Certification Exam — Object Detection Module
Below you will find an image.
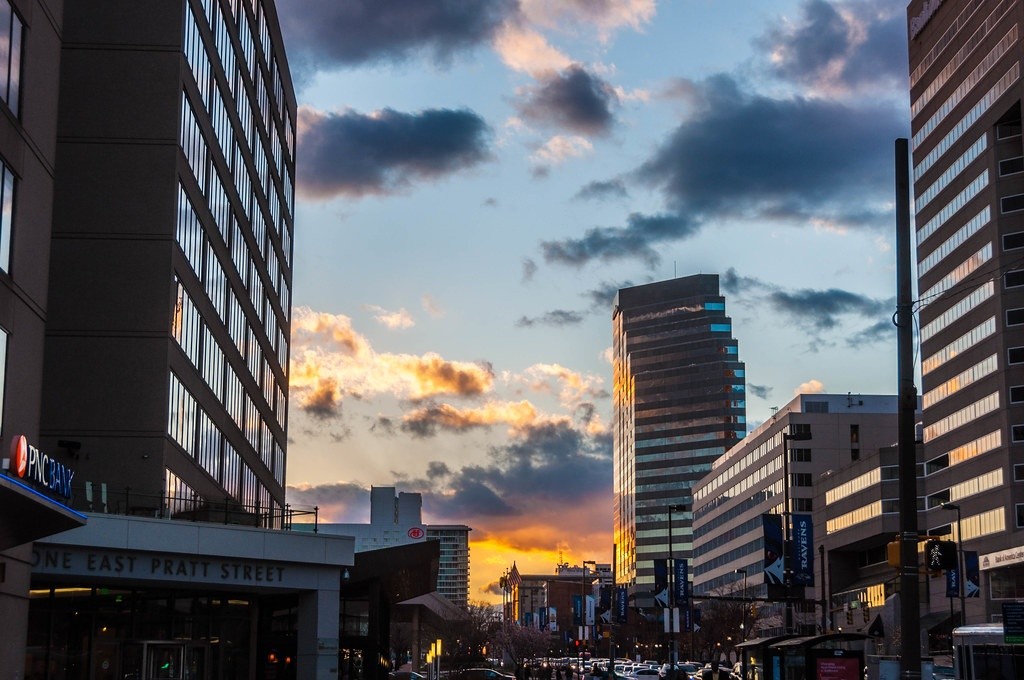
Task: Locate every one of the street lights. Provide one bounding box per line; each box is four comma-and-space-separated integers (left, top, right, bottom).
781, 430, 814, 632
431, 642, 436, 680
666, 505, 686, 680
436, 638, 442, 680
939, 503, 966, 626
735, 568, 748, 643
426, 653, 430, 680
581, 560, 596, 670
429, 649, 432, 680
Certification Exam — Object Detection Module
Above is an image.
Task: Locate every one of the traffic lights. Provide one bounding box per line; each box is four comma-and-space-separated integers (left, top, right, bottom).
925, 539, 951, 571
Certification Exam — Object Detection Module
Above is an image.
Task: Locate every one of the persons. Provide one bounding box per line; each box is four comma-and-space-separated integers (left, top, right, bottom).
514, 662, 601, 680
702, 661, 729, 680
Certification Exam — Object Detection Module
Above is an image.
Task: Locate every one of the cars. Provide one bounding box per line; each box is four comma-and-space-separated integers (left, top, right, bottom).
460, 667, 515, 680
488, 656, 764, 680
